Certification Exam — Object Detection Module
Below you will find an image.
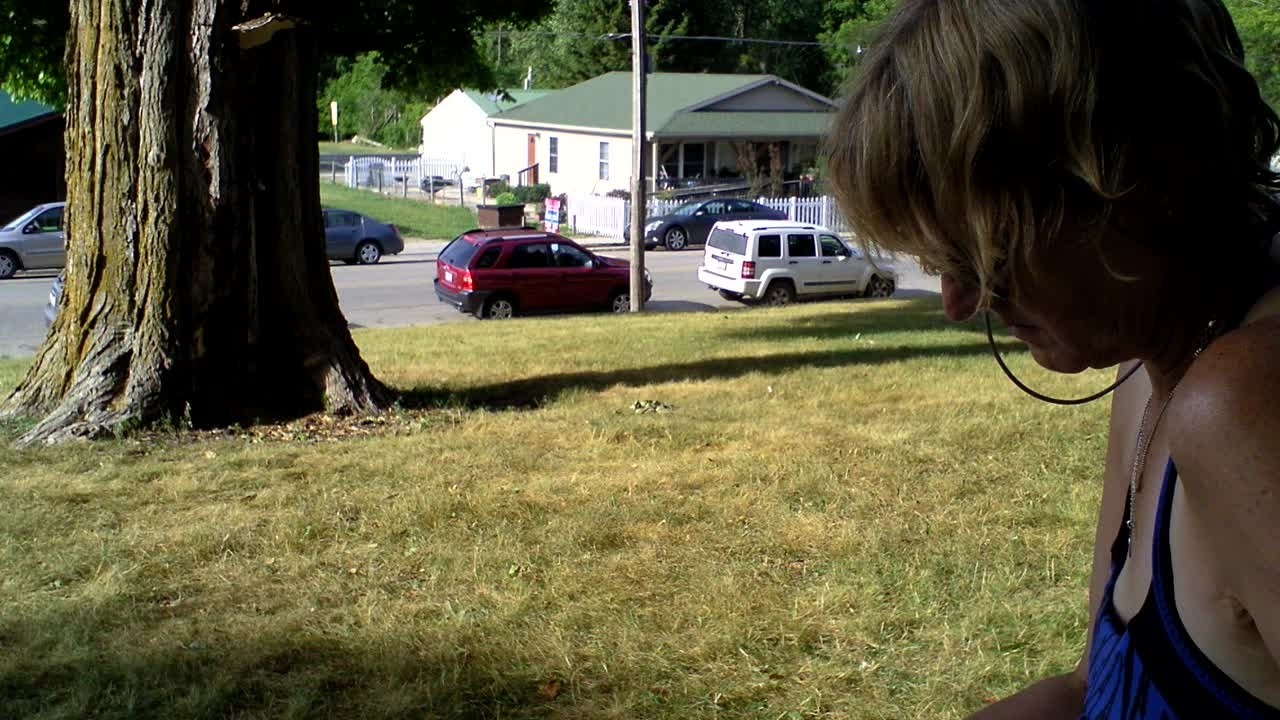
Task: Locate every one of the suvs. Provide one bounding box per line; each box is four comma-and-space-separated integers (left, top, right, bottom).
697, 219, 897, 306
0, 202, 67, 280
432, 225, 653, 320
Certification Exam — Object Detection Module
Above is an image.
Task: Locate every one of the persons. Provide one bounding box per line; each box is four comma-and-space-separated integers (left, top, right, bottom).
811, 0, 1280, 720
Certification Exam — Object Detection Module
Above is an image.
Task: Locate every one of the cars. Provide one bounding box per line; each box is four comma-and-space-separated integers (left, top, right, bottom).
322, 209, 403, 265
624, 199, 788, 251
